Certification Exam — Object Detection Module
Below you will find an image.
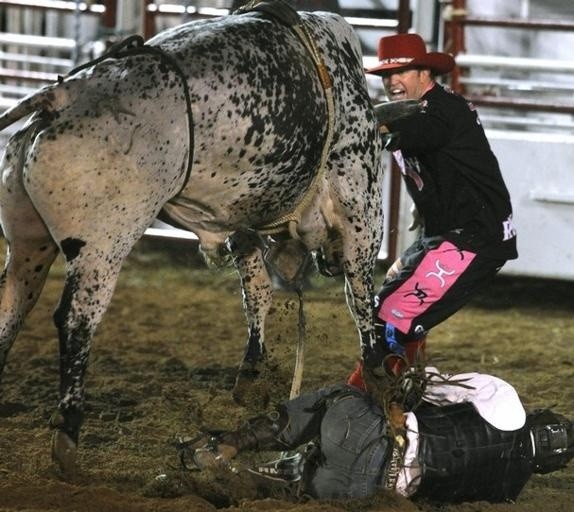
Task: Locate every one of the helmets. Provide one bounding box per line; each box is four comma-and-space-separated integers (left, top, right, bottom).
526, 409, 574, 475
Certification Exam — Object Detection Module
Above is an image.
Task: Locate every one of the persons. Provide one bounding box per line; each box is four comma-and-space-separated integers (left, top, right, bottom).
177, 358, 573, 508
343, 33, 518, 400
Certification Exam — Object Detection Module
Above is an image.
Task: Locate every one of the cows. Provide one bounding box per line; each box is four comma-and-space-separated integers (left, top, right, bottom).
0, 12, 385, 486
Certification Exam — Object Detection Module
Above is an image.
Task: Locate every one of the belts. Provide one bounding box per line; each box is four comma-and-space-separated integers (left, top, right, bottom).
376, 428, 407, 495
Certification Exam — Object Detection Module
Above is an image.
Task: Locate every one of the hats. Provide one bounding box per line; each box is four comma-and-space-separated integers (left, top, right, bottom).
366, 34, 456, 74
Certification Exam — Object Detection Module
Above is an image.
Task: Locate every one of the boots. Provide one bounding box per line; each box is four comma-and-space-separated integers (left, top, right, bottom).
148, 403, 294, 487
